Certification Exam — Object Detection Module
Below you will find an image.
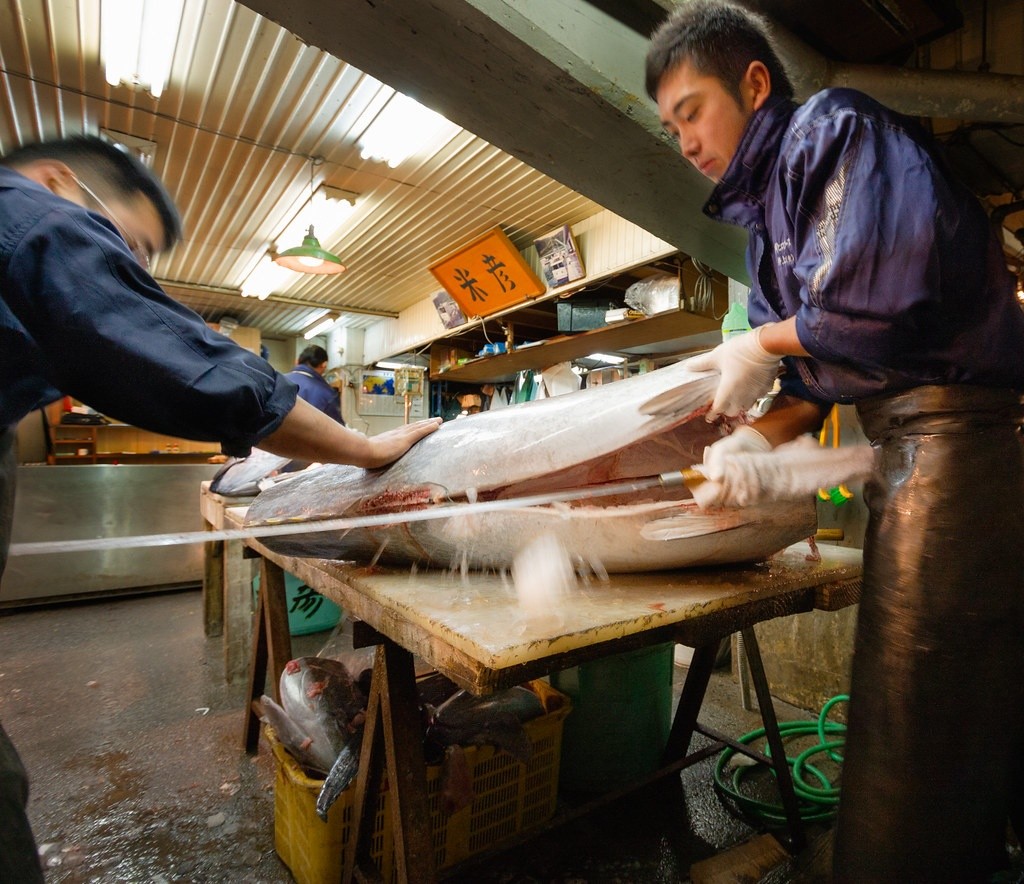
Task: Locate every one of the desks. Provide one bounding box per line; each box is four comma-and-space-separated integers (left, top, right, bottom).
222, 506, 866, 883
198, 478, 259, 687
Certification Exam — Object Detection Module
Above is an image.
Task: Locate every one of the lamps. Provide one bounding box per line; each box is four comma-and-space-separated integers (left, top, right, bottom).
217, 317, 240, 337
237, 184, 360, 301
300, 313, 341, 340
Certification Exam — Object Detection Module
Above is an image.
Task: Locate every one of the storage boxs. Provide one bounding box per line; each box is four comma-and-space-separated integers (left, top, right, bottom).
264, 666, 572, 884
253, 570, 344, 638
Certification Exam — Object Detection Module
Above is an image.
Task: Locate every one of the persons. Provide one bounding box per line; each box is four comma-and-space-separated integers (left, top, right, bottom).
642, 0, 1023, 882
0, 134, 443, 884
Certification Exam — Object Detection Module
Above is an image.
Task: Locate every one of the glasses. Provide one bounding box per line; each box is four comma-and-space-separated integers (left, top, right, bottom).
71, 175, 153, 275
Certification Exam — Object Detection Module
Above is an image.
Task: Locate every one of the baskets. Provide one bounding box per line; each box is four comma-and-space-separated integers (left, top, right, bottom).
265, 678, 574, 884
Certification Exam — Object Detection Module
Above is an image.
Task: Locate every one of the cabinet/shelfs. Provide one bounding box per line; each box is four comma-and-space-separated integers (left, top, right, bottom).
49, 396, 225, 467
428, 251, 734, 384
